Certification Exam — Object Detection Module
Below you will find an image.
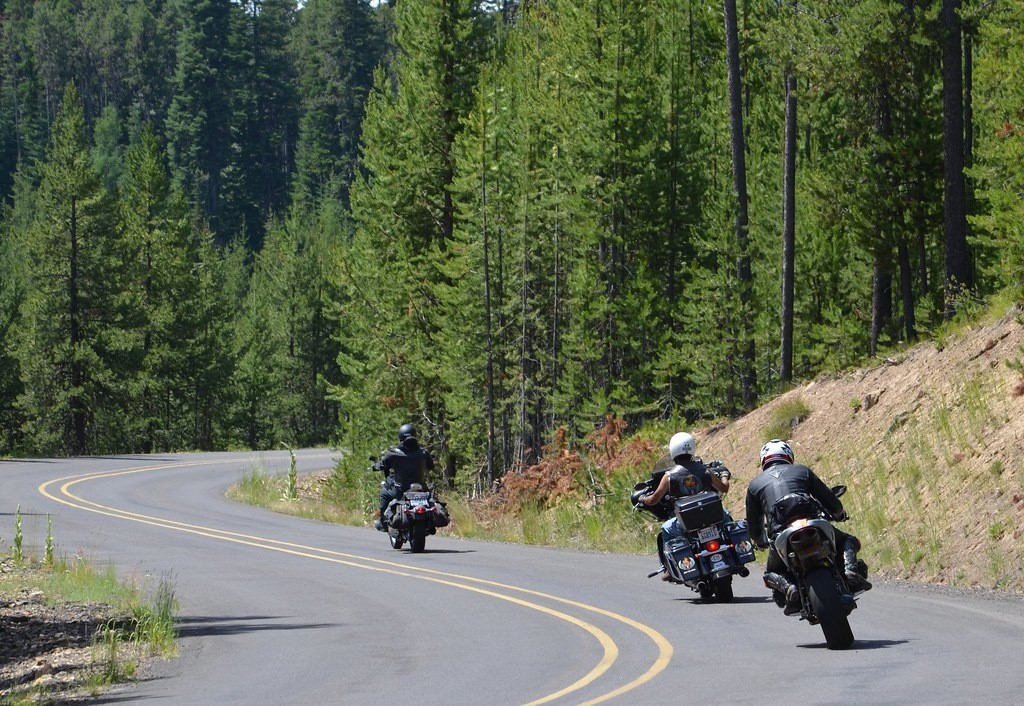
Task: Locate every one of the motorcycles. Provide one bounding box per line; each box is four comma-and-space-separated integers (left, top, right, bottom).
367, 454, 450, 553
752, 485, 873, 650
631, 454, 756, 601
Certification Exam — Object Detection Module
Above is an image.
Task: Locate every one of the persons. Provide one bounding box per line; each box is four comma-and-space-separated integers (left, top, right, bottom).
746, 439, 872, 616
637, 432, 732, 582
370, 424, 436, 535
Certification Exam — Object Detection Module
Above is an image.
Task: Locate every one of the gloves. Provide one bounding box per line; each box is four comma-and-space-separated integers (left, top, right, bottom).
717, 466, 730, 479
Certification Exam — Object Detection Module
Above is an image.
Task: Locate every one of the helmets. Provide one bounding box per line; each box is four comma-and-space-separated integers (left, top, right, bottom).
759, 439, 794, 471
397, 425, 417, 441
669, 432, 695, 460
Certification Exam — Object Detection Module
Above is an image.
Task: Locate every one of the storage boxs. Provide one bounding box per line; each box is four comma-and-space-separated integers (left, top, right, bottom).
664, 536, 700, 582
675, 490, 724, 530
725, 519, 756, 565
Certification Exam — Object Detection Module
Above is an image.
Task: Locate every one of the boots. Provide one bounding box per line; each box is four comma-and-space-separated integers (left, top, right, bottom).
766, 572, 802, 616
841, 550, 872, 593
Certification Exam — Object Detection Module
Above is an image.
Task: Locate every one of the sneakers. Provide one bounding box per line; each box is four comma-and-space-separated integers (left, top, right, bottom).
661, 572, 673, 581
375, 521, 388, 533
